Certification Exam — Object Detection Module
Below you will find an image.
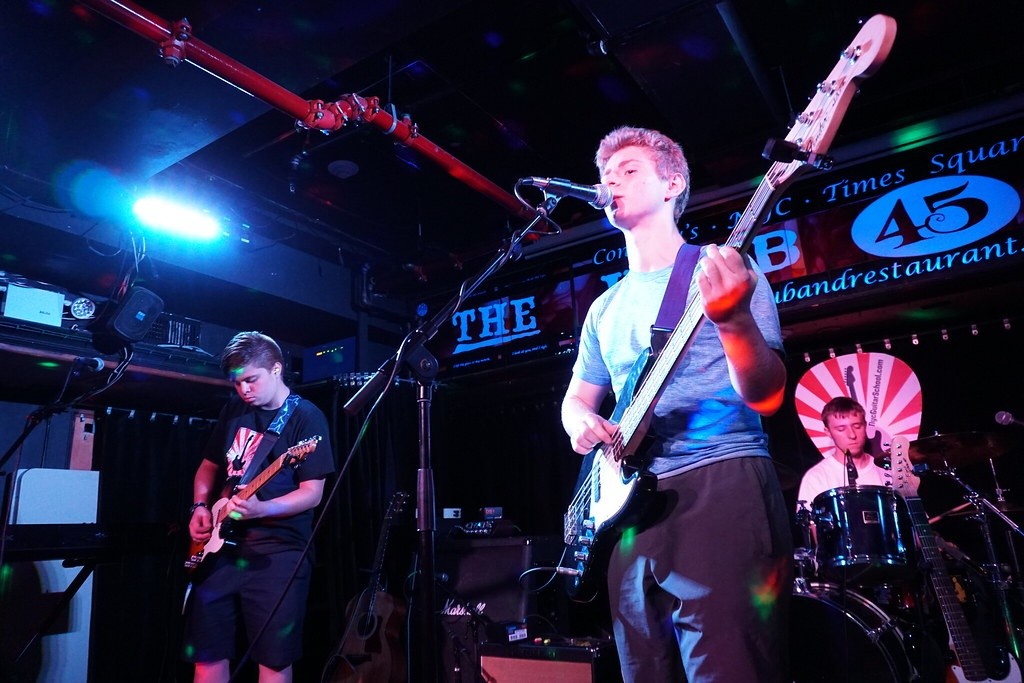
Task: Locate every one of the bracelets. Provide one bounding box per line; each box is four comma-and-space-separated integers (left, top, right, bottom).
189, 502, 209, 517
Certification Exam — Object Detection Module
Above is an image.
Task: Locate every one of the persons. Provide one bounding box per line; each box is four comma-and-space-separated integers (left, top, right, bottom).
188, 332, 334, 683
796, 396, 958, 560
561, 128, 787, 683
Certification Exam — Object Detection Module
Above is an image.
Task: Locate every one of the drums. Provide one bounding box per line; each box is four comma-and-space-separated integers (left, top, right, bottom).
812, 483, 912, 576
786, 582, 919, 683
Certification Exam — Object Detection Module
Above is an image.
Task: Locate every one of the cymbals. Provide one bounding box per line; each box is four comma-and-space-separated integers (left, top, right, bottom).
947, 499, 1023, 519
907, 428, 1023, 468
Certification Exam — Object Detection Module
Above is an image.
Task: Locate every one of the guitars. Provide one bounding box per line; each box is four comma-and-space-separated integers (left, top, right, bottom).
184, 435, 323, 575
321, 489, 411, 683
883, 441, 1023, 683
550, 13, 898, 605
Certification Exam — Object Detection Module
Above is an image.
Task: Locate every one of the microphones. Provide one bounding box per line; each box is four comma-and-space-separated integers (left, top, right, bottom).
845, 449, 858, 480
77, 356, 104, 372
995, 411, 1024, 427
519, 175, 613, 210
418, 569, 449, 583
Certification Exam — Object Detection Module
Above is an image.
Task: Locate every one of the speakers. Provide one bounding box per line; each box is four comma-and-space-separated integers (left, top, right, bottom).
433, 534, 565, 683
105, 286, 164, 344
478, 638, 624, 683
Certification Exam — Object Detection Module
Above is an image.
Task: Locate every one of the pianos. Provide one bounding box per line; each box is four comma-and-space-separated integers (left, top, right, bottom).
0, 523, 177, 564
